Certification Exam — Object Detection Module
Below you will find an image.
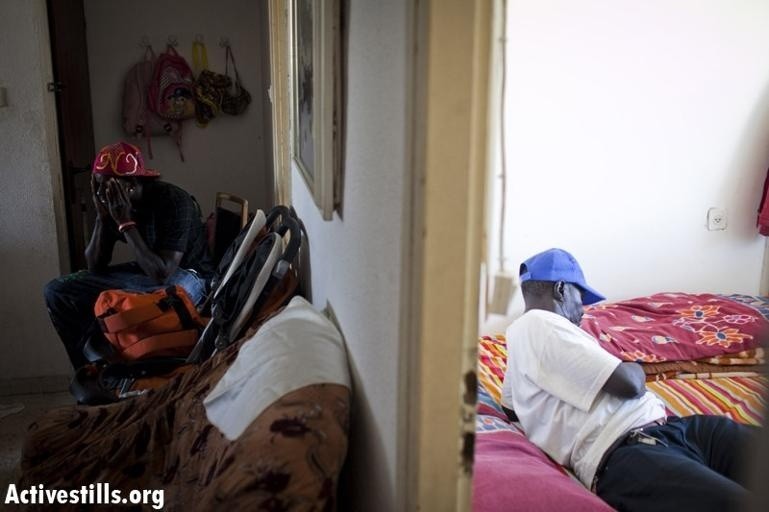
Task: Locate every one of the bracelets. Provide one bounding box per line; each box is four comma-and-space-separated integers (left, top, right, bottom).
118, 220, 138, 234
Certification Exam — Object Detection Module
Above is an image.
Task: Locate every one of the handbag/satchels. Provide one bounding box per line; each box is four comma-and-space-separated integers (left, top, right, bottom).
222, 87, 250, 114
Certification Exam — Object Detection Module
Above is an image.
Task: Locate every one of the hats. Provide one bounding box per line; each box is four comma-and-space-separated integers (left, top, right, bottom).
92, 142, 160, 176
519, 249, 606, 304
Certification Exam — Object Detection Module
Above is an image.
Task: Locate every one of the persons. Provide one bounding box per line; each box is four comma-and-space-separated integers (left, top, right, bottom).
499, 248, 769, 512
42, 140, 218, 377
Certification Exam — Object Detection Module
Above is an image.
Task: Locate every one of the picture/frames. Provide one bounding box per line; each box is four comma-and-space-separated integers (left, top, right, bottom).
292, 0, 341, 220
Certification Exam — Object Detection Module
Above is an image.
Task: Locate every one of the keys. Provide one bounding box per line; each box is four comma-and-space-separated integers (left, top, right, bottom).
626, 431, 657, 448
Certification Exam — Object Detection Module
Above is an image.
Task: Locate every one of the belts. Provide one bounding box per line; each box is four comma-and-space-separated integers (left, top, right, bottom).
592, 417, 666, 494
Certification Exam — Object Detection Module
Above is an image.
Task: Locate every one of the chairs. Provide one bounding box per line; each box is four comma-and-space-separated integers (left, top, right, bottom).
5, 304, 350, 511
214, 193, 249, 272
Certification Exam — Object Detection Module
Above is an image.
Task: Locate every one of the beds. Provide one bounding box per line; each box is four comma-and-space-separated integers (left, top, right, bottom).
473, 294, 769, 512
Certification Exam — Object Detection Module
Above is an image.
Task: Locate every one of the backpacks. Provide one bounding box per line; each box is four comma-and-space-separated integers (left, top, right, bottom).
149, 53, 196, 120
123, 44, 179, 137
95, 288, 205, 366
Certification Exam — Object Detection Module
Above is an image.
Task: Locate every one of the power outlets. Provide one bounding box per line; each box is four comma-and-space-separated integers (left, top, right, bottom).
708, 208, 727, 231
